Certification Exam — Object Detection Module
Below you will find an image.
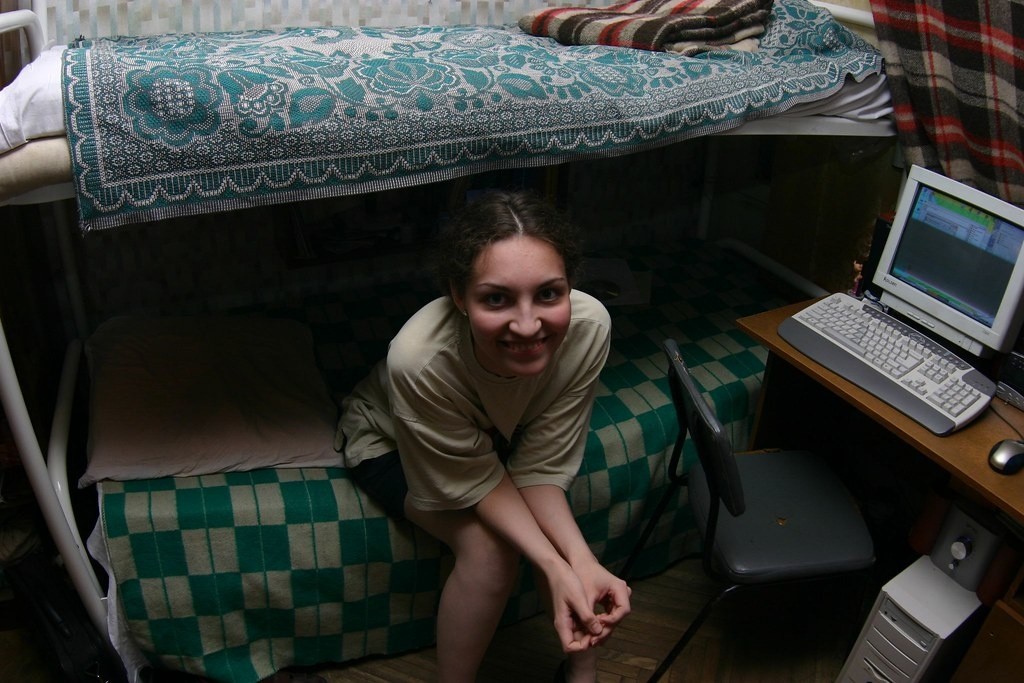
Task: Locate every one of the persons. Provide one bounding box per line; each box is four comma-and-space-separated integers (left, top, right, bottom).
330, 191, 632, 683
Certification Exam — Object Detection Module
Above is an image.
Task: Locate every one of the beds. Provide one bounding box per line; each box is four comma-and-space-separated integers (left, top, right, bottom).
0, 9, 894, 683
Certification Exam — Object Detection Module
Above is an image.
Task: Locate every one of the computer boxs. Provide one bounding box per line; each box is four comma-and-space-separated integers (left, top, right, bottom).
835, 553, 988, 683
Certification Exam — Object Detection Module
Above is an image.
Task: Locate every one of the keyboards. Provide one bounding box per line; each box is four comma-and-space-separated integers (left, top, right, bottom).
778, 293, 997, 437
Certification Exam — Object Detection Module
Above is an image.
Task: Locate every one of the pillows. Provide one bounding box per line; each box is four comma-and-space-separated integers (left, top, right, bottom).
78, 314, 346, 489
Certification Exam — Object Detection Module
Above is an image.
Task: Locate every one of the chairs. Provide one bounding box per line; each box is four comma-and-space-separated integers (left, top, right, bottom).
618, 341, 879, 683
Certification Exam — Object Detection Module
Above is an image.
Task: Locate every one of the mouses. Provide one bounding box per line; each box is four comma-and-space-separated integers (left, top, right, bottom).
987, 438, 1024, 475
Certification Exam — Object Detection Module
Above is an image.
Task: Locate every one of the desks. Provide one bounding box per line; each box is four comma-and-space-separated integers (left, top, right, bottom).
733, 289, 1024, 683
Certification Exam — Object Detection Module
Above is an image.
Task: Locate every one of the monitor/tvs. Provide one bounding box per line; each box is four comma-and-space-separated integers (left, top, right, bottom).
871, 164, 1024, 357
